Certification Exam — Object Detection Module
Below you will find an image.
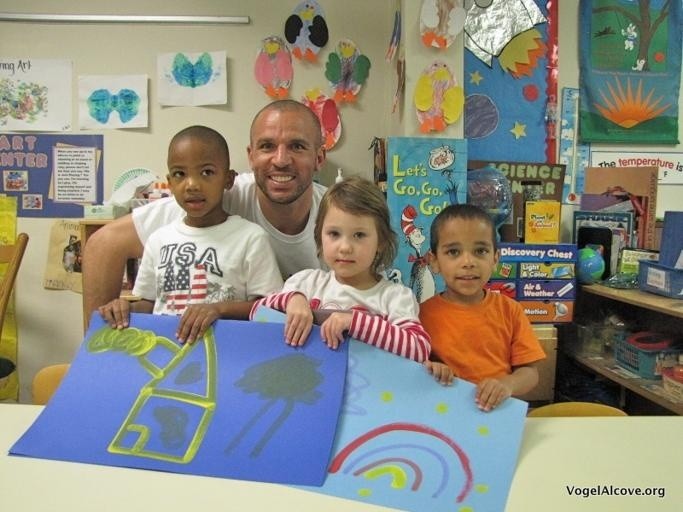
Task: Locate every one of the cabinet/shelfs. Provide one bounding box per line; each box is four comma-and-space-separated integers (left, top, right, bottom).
552, 272, 682, 415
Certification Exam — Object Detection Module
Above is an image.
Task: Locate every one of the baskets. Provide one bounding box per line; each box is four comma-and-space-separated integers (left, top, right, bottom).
611, 329, 683, 404
636, 259, 683, 299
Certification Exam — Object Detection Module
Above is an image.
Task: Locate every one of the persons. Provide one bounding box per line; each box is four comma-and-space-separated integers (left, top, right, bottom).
416, 204, 549, 414
246, 173, 432, 365
77, 100, 390, 342
97, 123, 285, 345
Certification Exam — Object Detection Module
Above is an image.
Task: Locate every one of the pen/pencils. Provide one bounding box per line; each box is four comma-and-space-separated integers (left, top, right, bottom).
130, 174, 159, 200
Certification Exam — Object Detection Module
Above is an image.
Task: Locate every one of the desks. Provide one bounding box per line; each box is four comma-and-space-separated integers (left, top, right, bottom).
1, 400, 683, 511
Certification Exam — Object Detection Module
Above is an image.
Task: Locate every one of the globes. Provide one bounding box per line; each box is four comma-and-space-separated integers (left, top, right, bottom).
574, 244, 605, 285
